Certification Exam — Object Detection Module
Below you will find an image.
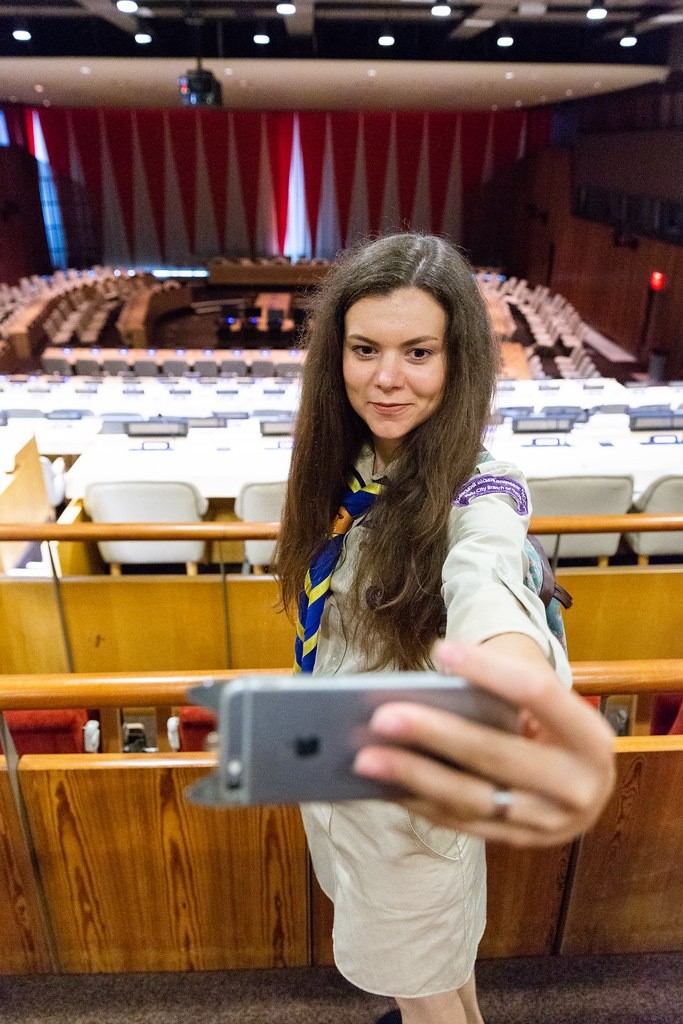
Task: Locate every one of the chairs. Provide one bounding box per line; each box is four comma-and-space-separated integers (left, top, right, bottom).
0, 256, 683, 575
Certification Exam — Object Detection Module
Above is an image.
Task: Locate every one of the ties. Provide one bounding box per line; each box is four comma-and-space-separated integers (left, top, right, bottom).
294, 468, 395, 674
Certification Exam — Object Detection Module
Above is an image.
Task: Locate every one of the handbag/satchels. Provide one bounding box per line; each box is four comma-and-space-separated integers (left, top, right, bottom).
525, 530, 571, 649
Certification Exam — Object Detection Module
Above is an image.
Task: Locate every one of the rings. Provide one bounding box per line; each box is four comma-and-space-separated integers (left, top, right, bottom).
489, 785, 509, 824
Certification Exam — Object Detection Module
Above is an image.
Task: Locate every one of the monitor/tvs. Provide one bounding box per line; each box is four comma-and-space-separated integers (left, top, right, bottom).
220, 303, 306, 325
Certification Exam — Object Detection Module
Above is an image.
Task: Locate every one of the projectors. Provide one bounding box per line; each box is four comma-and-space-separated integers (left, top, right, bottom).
178, 70, 222, 108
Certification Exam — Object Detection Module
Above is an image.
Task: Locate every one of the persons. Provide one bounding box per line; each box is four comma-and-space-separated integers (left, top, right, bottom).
273, 235, 618, 1024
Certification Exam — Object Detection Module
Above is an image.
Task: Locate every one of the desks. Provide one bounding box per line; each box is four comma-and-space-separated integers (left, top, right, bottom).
500, 342, 532, 380
115, 283, 192, 349
481, 293, 509, 334
8, 297, 56, 359
208, 264, 334, 284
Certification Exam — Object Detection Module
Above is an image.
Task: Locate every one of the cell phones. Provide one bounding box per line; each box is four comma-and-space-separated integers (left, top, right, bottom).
186, 673, 520, 810
